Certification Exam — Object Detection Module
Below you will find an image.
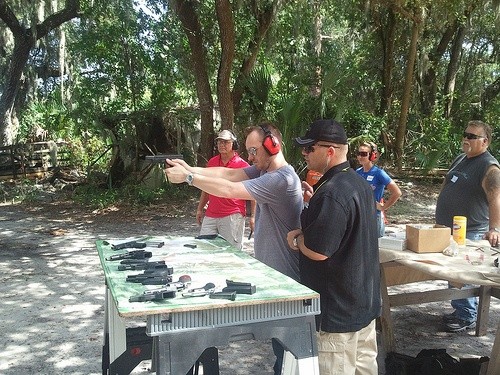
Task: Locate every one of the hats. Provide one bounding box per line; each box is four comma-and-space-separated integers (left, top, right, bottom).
295, 120, 348, 144
215, 130, 236, 142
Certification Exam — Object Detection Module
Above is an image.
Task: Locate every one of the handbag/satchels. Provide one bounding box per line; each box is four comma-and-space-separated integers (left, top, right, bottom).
386, 348, 463, 375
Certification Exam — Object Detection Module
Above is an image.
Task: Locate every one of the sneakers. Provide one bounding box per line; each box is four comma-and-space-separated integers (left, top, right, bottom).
443, 312, 457, 321
448, 319, 476, 333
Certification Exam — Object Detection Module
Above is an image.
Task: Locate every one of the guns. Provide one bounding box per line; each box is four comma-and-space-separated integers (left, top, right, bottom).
146, 153, 184, 166
106, 240, 258, 303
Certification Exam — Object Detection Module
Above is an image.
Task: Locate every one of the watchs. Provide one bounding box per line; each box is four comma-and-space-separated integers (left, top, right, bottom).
186, 173, 193, 186
293, 234, 301, 249
489, 228, 500, 232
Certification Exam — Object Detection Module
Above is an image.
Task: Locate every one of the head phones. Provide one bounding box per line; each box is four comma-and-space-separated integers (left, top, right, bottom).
214, 129, 240, 151
258, 125, 281, 156
367, 143, 380, 162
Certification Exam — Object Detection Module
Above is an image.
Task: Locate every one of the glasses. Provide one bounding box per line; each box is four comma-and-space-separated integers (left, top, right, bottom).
247, 144, 263, 156
217, 141, 232, 145
305, 144, 340, 153
463, 132, 487, 139
357, 152, 371, 157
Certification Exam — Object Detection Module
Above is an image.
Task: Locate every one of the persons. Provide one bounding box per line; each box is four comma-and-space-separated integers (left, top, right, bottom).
304, 170, 323, 207
356, 141, 403, 239
196, 130, 256, 251
436, 120, 500, 333
163, 120, 304, 375
286, 120, 383, 375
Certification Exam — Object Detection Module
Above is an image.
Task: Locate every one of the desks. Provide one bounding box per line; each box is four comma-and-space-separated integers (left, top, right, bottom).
95, 233, 322, 375
379, 222, 500, 375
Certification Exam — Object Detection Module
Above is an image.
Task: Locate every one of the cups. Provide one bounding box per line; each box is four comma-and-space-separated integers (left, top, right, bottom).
453, 216, 467, 248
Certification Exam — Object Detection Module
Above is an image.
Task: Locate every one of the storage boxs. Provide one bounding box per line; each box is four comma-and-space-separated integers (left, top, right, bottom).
378, 223, 451, 253
